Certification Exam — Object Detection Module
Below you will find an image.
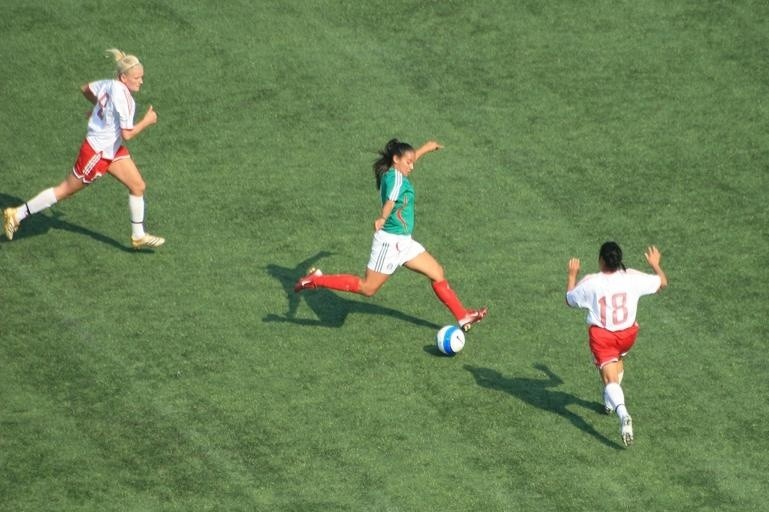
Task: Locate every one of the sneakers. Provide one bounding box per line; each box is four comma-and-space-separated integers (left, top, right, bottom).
603, 402, 615, 415
293, 267, 322, 292
4, 207, 20, 240
130, 233, 165, 251
457, 307, 488, 332
620, 415, 634, 446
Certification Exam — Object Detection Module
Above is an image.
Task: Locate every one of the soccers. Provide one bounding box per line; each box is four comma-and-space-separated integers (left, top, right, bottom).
436, 325, 465, 357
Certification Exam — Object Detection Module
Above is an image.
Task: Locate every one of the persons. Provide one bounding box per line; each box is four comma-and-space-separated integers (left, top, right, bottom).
564, 240, 669, 447
294, 139, 488, 333
3, 47, 167, 249
